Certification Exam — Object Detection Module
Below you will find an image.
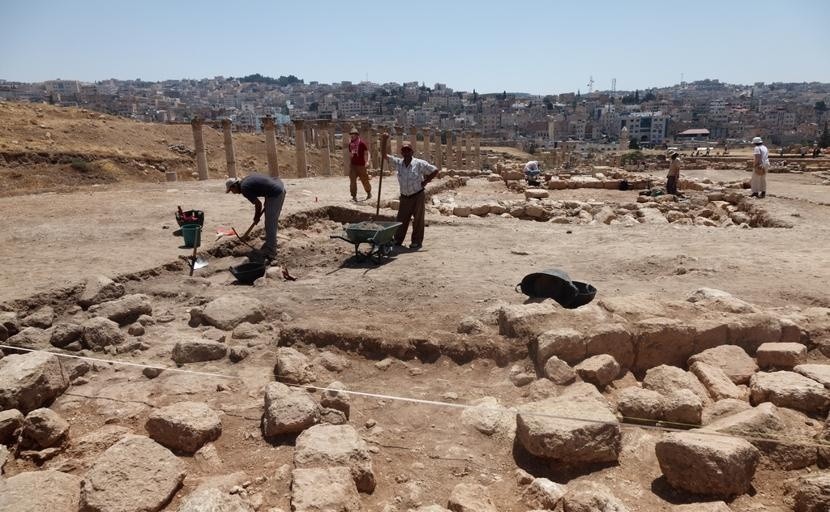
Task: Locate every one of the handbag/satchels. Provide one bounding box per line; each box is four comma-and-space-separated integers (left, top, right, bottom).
755, 162, 765, 175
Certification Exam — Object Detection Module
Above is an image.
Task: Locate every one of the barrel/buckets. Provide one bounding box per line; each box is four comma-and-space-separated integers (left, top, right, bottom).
182, 224, 201, 248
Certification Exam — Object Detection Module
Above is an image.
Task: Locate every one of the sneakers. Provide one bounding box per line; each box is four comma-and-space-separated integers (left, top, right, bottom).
349, 193, 372, 202
389, 239, 400, 246
410, 242, 422, 250
749, 192, 765, 198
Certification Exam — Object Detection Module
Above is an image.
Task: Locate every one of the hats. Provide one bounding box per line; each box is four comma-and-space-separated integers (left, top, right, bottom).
668, 149, 676, 156
750, 137, 763, 145
226, 178, 239, 194
349, 128, 360, 136
401, 144, 414, 151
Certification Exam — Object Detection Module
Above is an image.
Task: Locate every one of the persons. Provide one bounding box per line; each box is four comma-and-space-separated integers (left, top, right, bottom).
748, 137, 770, 198
690, 143, 822, 160
347, 127, 372, 202
226, 174, 286, 260
381, 129, 439, 250
665, 149, 682, 195
523, 160, 541, 181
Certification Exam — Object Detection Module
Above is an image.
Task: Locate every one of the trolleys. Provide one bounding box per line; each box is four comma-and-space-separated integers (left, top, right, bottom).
329, 220, 404, 265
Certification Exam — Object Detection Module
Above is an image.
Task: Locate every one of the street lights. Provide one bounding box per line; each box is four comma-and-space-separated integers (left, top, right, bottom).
500, 110, 505, 127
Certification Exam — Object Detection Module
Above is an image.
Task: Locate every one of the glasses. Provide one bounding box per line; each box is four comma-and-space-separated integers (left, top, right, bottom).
402, 149, 411, 152
